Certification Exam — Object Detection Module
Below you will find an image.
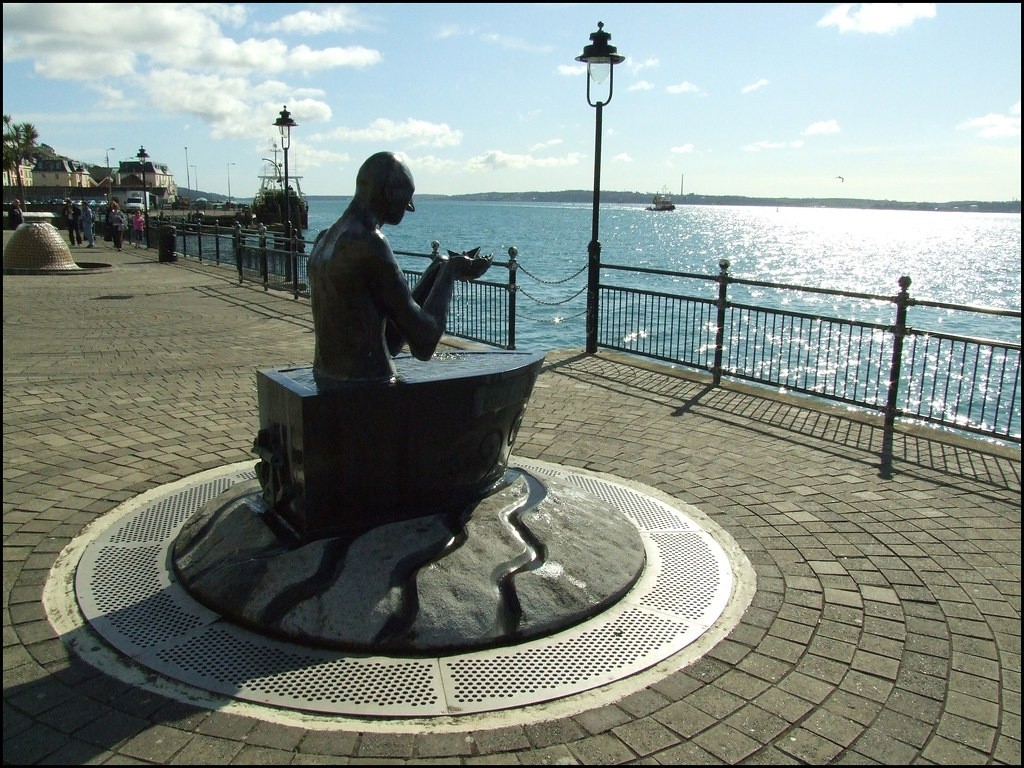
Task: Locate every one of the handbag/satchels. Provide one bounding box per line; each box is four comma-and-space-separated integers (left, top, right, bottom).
118, 224, 128, 231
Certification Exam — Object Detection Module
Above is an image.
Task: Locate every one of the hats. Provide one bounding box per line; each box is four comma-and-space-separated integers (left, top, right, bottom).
64, 199, 71, 204
82, 201, 89, 206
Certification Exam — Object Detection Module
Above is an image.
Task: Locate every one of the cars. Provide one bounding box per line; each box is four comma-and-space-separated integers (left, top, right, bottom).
95, 200, 107, 206
87, 199, 97, 206
71, 199, 82, 205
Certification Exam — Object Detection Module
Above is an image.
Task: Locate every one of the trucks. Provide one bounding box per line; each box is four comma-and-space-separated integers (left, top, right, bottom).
123, 191, 150, 214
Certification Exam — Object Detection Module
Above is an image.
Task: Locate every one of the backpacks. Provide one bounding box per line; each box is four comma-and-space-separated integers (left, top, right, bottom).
88, 207, 96, 223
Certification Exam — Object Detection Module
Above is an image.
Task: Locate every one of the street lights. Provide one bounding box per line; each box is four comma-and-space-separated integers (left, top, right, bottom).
227, 162, 236, 205
273, 103, 302, 282
575, 19, 625, 355
106, 147, 115, 199
135, 145, 150, 248
189, 164, 198, 196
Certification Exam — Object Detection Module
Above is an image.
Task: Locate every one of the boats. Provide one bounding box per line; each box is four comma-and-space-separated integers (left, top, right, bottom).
644, 192, 677, 211
248, 141, 310, 230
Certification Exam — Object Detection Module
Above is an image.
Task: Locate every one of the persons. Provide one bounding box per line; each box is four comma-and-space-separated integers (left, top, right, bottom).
62, 197, 143, 253
9, 199, 23, 230
306, 151, 493, 383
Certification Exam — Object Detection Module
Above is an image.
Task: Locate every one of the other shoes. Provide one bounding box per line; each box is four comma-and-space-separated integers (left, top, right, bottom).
118, 248, 121, 252
69, 244, 76, 247
78, 244, 82, 248
86, 245, 95, 248
